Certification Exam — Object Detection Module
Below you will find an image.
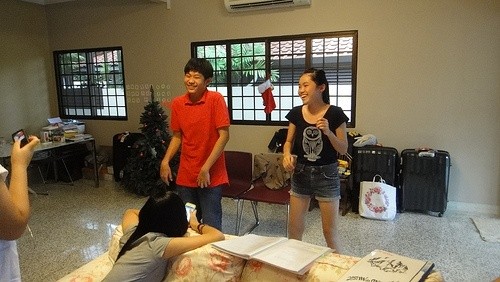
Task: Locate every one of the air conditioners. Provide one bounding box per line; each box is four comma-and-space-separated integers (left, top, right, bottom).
224, 0, 311, 13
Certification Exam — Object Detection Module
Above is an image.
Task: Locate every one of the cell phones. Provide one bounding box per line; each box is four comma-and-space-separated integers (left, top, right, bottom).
185, 202, 197, 223
11, 129, 29, 148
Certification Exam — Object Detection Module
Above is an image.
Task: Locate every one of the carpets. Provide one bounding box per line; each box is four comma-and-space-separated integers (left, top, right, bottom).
470, 216, 500, 243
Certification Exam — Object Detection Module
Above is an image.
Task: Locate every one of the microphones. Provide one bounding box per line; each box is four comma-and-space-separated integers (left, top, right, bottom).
250, 172, 268, 186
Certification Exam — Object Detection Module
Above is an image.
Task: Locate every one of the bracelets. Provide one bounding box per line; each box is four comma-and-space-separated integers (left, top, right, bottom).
197, 224, 205, 234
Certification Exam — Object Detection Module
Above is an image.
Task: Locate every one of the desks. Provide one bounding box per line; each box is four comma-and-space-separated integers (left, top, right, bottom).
0, 134, 100, 188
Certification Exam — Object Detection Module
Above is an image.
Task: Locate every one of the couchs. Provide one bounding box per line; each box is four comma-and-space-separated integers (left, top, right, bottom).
59, 226, 446, 282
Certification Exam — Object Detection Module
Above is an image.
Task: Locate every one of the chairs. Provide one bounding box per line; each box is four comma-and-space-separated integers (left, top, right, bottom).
221, 129, 362, 239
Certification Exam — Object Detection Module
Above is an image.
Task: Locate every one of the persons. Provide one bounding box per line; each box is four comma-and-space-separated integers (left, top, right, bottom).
282, 68, 349, 253
102, 190, 225, 282
160, 58, 231, 233
0, 135, 41, 282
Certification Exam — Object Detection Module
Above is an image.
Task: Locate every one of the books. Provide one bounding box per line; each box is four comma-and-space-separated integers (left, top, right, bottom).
339, 249, 436, 282
212, 233, 335, 276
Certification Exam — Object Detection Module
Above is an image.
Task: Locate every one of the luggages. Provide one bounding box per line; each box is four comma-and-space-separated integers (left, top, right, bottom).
112, 133, 146, 180
400, 148, 451, 216
350, 146, 402, 213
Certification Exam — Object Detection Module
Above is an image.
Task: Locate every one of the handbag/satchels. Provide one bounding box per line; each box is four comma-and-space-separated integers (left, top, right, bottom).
359, 174, 398, 221
